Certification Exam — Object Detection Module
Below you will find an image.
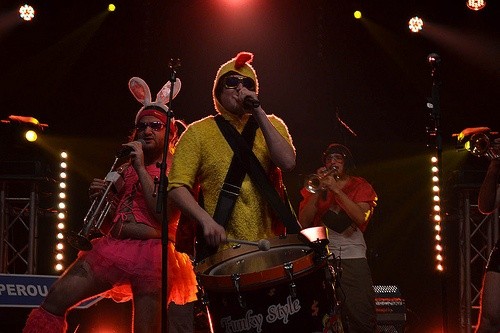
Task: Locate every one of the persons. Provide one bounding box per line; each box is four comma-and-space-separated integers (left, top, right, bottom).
22, 102, 198, 333
478, 138, 500, 333
167, 52, 295, 267
299, 144, 378, 333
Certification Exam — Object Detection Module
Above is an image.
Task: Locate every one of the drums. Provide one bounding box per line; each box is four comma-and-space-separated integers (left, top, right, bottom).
192, 234, 346, 333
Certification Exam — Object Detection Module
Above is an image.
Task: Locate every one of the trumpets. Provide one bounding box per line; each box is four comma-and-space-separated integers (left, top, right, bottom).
65, 127, 140, 252
303, 166, 341, 193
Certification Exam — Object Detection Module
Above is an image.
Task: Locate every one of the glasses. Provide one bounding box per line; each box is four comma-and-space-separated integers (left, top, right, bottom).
135, 122, 165, 131
325, 156, 345, 164
222, 76, 255, 90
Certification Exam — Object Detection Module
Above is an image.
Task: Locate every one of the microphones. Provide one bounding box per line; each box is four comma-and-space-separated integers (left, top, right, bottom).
243, 95, 260, 108
427, 53, 440, 65
